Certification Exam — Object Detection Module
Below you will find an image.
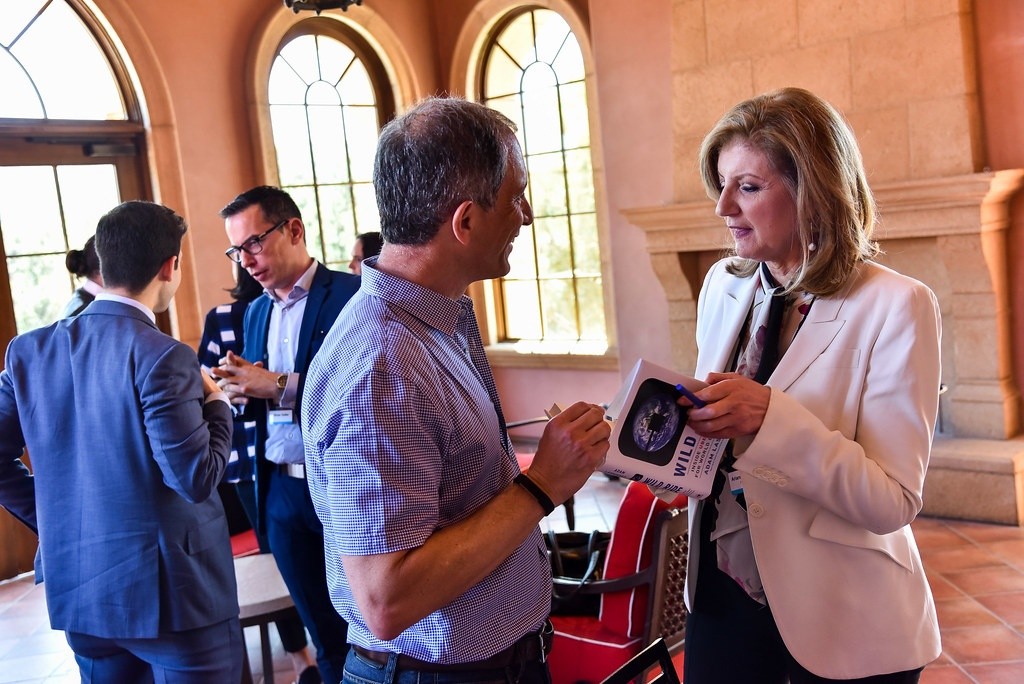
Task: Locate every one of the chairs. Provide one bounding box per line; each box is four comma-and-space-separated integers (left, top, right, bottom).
546, 476, 691, 684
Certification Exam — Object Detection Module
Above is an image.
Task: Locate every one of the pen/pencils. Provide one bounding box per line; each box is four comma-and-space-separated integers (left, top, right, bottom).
676, 382, 706, 409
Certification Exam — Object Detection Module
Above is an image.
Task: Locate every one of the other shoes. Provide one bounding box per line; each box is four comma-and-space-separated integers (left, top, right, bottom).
299, 666, 322, 684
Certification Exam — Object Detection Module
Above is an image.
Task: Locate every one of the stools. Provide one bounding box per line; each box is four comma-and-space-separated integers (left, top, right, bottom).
224, 554, 298, 684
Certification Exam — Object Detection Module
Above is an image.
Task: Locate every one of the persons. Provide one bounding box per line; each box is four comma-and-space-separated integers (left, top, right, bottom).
65, 185, 389, 684
0, 201, 244, 684
301, 95, 611, 684
647, 87, 943, 684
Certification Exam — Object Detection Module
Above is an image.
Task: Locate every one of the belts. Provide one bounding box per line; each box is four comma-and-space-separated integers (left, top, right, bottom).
282, 463, 307, 479
354, 618, 554, 672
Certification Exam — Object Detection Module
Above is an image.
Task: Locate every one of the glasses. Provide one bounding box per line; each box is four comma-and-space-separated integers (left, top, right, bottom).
226, 220, 287, 263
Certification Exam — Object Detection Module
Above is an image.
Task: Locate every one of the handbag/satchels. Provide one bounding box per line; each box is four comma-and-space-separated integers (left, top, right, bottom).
541, 531, 611, 617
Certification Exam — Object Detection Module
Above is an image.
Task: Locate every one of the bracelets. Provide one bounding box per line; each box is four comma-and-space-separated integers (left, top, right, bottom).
513, 474, 555, 516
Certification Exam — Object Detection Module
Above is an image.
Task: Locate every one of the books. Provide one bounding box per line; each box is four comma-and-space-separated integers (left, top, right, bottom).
544, 357, 730, 500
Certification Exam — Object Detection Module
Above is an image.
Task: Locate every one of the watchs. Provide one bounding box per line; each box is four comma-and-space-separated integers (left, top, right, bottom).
277, 373, 288, 398
